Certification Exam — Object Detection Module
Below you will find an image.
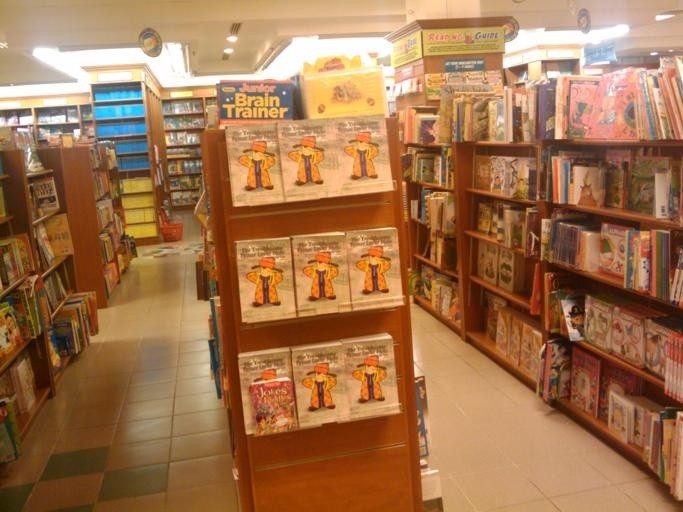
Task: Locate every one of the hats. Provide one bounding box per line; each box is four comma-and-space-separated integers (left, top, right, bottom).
307, 363, 336, 379
568, 305, 585, 317
348, 131, 379, 148
356, 355, 387, 372
244, 141, 275, 156
306, 252, 338, 267
252, 257, 284, 272
360, 246, 391, 262
292, 136, 324, 151
253, 369, 279, 382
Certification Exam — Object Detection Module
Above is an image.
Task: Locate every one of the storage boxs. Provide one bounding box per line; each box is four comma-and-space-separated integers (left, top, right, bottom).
296, 64, 389, 120
216, 79, 295, 130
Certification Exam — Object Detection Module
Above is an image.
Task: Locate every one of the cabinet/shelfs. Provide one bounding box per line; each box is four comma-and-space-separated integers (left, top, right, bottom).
91, 79, 170, 245
550, 84, 683, 501
0, 91, 94, 142
134, 82, 203, 246
26, 139, 131, 309
465, 99, 548, 390
396, 112, 465, 328
0, 147, 91, 443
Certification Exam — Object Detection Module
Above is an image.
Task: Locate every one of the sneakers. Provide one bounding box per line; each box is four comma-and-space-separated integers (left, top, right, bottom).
308, 296, 320, 303
327, 405, 336, 409
308, 406, 318, 412
351, 174, 361, 180
313, 179, 324, 185
369, 174, 378, 179
245, 184, 254, 192
358, 397, 367, 404
362, 289, 371, 295
376, 397, 386, 403
252, 302, 262, 306
271, 301, 282, 307
327, 294, 336, 300
263, 184, 275, 191
380, 288, 389, 293
295, 179, 305, 186
